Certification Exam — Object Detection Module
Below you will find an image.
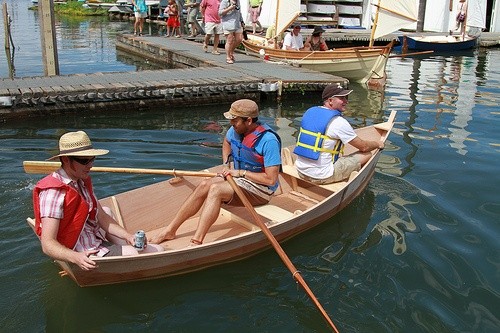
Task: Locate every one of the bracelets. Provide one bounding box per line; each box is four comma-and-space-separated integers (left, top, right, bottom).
243, 168, 248, 177
238, 169, 241, 177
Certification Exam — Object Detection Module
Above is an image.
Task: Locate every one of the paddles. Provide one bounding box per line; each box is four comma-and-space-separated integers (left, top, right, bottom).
333, 45, 390, 51
388, 50, 434, 59
21, 160, 226, 178
223, 167, 340, 333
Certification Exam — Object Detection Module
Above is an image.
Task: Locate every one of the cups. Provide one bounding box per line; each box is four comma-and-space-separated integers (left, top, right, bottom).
265, 54, 269, 60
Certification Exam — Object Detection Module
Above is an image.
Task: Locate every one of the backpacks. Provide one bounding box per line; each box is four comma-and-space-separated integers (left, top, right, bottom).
168, 5, 178, 16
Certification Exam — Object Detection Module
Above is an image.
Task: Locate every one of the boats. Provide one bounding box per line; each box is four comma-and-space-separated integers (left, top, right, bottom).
242, 12, 396, 84
398, 25, 481, 52
26, 108, 398, 287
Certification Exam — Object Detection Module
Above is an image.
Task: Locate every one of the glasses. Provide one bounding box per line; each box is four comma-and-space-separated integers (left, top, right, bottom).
69, 156, 96, 165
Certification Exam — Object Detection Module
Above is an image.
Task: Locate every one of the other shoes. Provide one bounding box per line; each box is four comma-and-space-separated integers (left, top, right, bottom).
251, 32, 256, 35
196, 33, 204, 37
139, 33, 143, 37
188, 34, 194, 38
203, 43, 208, 52
212, 50, 220, 55
133, 31, 137, 36
229, 54, 235, 62
180, 34, 185, 36
170, 34, 174, 37
259, 28, 264, 35
164, 34, 169, 38
226, 57, 233, 64
175, 34, 181, 38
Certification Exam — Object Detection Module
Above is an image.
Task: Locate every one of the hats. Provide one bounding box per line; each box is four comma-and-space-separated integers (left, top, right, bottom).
312, 26, 326, 34
321, 83, 353, 101
223, 99, 258, 119
45, 130, 109, 160
293, 23, 302, 30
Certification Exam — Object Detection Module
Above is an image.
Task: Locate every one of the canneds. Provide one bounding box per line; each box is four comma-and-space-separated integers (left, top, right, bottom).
135, 230, 145, 251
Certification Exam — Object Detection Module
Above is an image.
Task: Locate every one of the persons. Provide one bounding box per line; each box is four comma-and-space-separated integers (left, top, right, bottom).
218, 0, 246, 64
281, 23, 330, 52
248, 0, 265, 35
292, 83, 385, 185
148, 99, 282, 244
33, 130, 147, 273
131, 0, 222, 55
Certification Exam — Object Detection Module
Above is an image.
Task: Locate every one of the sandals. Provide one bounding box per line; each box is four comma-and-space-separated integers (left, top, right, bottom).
186, 238, 202, 247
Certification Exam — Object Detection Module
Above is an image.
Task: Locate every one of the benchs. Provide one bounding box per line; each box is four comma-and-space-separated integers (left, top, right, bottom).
183, 176, 294, 223
283, 165, 347, 192
